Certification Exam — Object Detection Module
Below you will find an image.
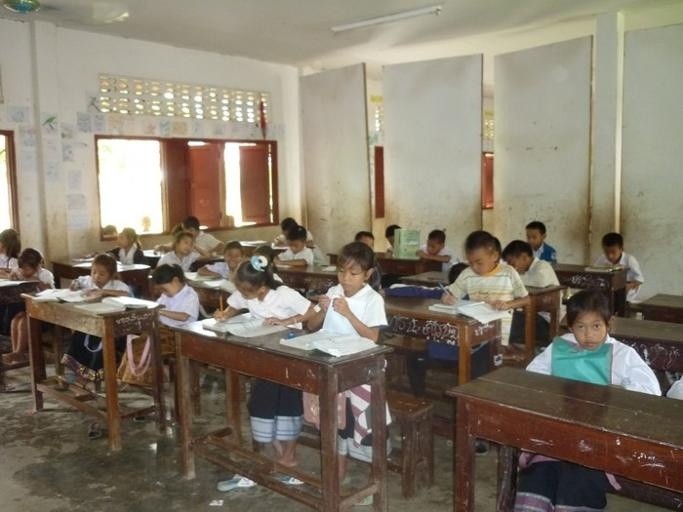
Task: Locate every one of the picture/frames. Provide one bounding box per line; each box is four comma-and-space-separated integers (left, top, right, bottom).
20, 291, 170, 453
0, 281, 39, 391
53, 253, 151, 299
444, 366, 682, 512
609, 315, 683, 373
238, 240, 263, 255
554, 262, 630, 315
400, 270, 451, 289
325, 250, 385, 268
143, 249, 161, 264
172, 317, 395, 511
636, 294, 682, 322
276, 266, 338, 294
377, 296, 501, 386
378, 257, 442, 276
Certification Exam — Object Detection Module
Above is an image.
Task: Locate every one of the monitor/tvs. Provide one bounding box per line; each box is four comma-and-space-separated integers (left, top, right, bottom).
584, 263, 623, 273
74, 296, 147, 315
427, 299, 510, 325
279, 328, 378, 358
202, 312, 290, 338
34, 289, 97, 302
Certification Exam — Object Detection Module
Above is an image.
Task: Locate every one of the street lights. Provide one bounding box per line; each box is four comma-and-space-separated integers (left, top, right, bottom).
551, 335, 613, 386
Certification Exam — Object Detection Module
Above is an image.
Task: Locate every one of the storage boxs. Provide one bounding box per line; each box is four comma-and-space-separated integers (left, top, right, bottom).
503, 341, 534, 370
387, 388, 435, 499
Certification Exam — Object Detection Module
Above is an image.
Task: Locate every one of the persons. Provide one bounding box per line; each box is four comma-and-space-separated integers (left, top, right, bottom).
392, 228, 421, 259
385, 262, 470, 398
106, 228, 146, 265
354, 230, 375, 252
196, 241, 243, 278
501, 239, 562, 342
213, 257, 316, 468
513, 290, 662, 512
273, 225, 314, 268
61, 254, 134, 383
2, 248, 56, 362
595, 232, 644, 304
525, 221, 558, 264
153, 225, 183, 254
439, 230, 530, 457
181, 216, 225, 256
306, 243, 393, 507
415, 229, 453, 273
385, 224, 402, 251
273, 216, 325, 264
116, 262, 200, 387
0, 229, 19, 280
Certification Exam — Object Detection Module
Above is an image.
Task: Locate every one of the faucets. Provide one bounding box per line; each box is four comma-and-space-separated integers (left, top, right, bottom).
87, 422, 102, 439
135, 413, 146, 422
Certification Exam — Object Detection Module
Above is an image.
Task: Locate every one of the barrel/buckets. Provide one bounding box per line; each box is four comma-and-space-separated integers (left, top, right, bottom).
358, 498, 373, 506
270, 471, 302, 485
217, 476, 256, 492
336, 474, 350, 495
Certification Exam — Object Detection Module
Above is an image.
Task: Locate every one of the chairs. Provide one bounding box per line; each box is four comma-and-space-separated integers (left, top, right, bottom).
476, 440, 489, 455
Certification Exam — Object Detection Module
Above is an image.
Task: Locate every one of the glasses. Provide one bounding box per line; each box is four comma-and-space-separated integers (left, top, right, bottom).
330, 4, 444, 35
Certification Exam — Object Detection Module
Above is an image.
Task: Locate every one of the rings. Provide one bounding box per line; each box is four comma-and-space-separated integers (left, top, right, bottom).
220, 295, 222, 312
438, 282, 458, 303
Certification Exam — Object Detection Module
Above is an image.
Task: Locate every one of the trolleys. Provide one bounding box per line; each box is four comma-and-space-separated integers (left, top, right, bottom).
0, 0, 61, 21
